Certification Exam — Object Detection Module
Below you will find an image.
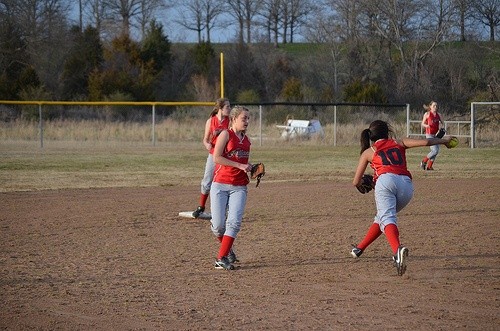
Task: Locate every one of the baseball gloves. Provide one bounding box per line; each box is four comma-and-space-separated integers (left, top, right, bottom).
247, 162, 266, 180
356, 175, 378, 194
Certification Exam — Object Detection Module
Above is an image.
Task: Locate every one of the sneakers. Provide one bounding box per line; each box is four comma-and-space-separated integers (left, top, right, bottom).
192, 207, 204, 218
228, 251, 235, 263
215, 257, 234, 270
350, 247, 363, 258
392, 246, 409, 275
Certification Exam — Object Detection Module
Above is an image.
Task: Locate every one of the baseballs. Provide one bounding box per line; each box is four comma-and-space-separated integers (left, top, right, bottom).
435, 128, 446, 138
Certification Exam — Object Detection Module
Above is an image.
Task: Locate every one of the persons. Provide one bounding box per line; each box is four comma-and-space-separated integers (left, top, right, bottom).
348, 119, 458, 275
192, 99, 232, 218
210, 105, 265, 270
421, 100, 445, 170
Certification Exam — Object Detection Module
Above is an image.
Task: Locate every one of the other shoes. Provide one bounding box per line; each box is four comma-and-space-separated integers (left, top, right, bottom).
421, 161, 427, 170
427, 167, 433, 170
